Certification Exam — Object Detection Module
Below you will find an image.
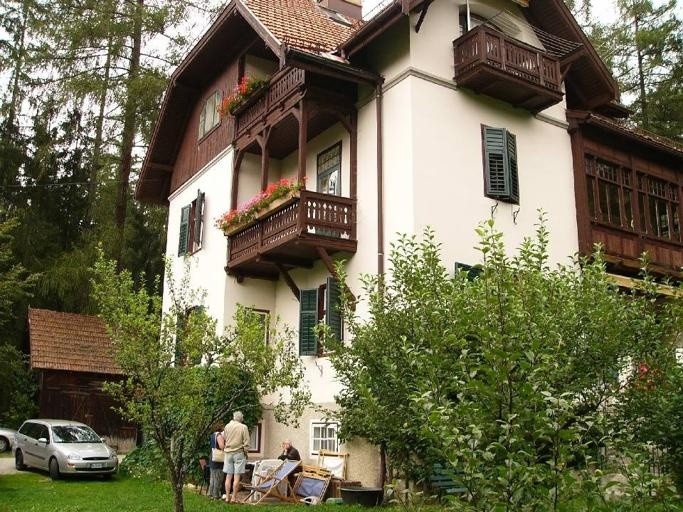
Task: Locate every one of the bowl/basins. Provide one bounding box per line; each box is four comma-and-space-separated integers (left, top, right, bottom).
339, 486, 384, 508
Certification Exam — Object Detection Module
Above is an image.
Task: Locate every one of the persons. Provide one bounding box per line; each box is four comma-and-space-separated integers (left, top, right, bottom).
276, 439, 302, 496
220, 410, 251, 504
206, 419, 226, 498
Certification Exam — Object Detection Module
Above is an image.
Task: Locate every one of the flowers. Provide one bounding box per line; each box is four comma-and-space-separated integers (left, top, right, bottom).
214, 71, 255, 118
214, 176, 299, 231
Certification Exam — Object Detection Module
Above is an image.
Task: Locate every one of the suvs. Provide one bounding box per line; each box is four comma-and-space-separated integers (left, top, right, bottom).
12, 417, 121, 484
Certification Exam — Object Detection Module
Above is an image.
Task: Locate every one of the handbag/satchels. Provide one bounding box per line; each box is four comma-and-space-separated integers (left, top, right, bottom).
210, 432, 225, 463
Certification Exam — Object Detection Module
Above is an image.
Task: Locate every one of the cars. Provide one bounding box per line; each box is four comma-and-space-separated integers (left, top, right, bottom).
0, 426, 19, 454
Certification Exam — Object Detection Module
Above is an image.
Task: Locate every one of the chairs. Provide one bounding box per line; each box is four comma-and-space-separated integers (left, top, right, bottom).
241, 458, 303, 505
